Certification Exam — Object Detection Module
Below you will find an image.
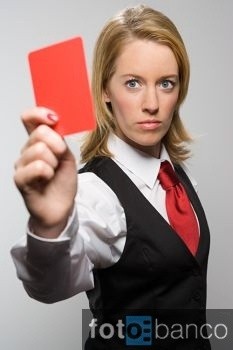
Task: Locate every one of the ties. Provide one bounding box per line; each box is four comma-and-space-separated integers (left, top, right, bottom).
157, 161, 199, 259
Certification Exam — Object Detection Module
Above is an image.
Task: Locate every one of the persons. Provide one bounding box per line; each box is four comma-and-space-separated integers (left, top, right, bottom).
11, 5, 212, 345
